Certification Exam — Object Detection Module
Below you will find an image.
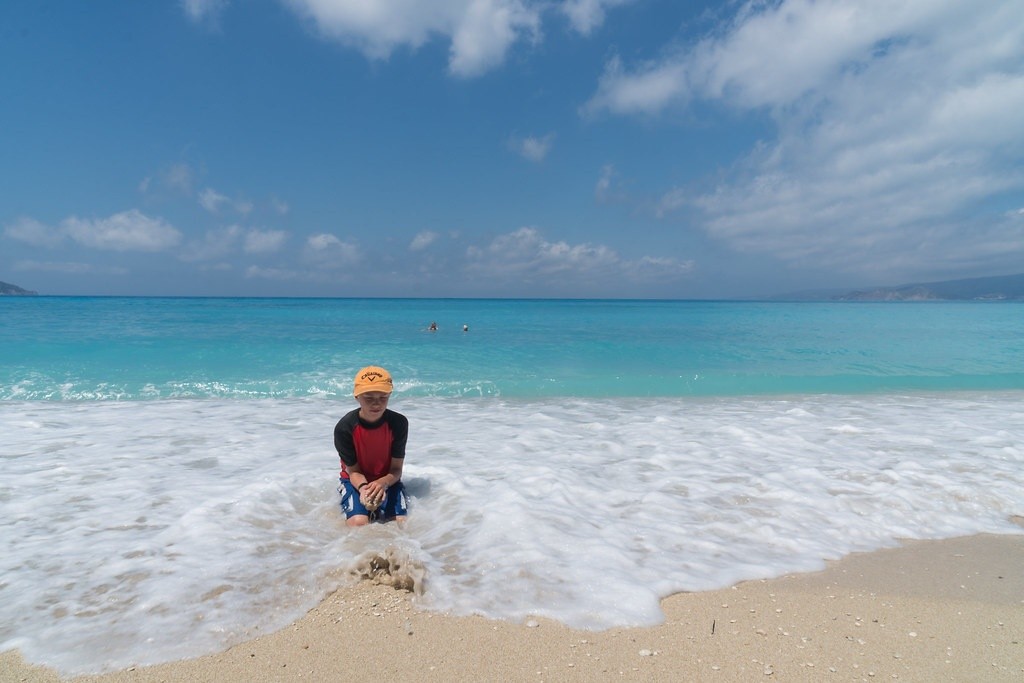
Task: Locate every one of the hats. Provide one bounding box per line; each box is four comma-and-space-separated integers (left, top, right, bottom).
354, 365, 393, 396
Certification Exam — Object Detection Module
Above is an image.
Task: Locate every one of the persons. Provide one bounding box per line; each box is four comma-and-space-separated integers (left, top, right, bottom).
334, 365, 409, 527
429, 321, 468, 331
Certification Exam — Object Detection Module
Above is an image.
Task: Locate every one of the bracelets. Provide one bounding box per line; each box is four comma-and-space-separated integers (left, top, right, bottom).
357, 483, 368, 491
